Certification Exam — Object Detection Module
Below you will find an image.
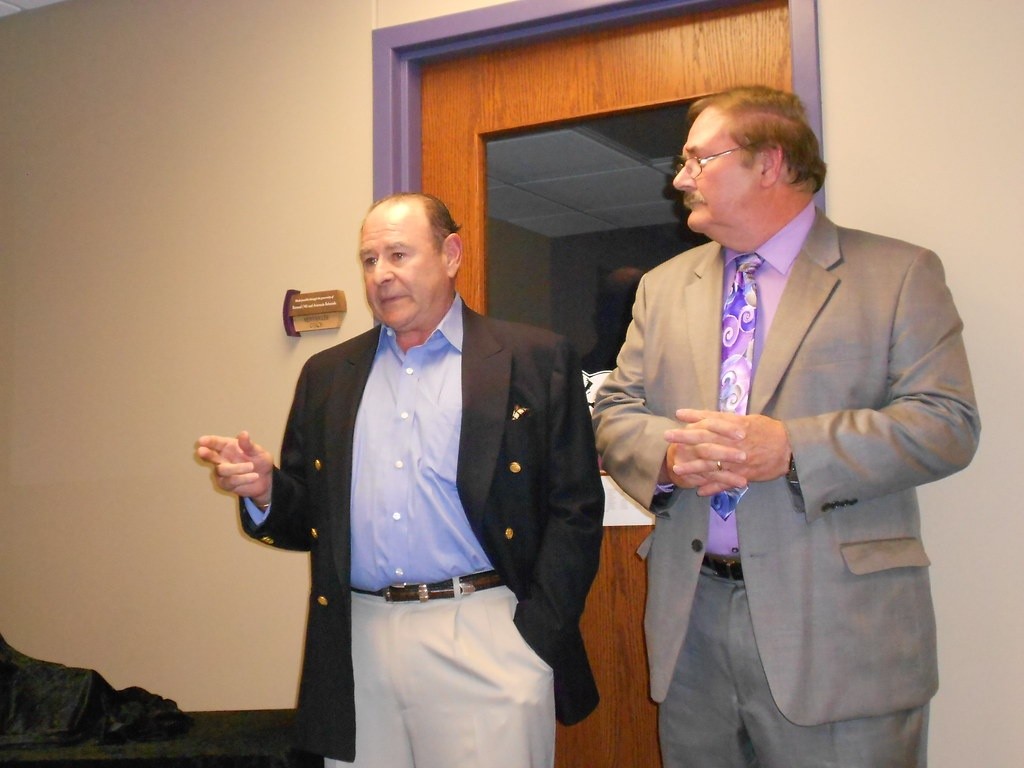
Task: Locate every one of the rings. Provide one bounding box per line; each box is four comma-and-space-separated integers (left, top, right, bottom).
717, 461, 722, 471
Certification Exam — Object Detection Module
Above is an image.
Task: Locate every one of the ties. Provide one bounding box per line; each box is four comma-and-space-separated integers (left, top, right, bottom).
708, 253, 765, 522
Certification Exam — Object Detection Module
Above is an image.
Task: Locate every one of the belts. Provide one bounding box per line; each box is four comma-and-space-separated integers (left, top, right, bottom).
349, 569, 505, 603
702, 555, 742, 582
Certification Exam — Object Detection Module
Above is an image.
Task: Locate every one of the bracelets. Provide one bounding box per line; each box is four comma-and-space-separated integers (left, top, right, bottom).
255, 502, 272, 509
789, 452, 798, 483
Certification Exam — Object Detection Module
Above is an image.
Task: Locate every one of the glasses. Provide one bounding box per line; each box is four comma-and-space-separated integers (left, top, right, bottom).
674, 138, 770, 181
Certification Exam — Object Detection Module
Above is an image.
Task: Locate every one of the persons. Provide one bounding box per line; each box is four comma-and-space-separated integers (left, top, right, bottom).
197, 195, 605, 768
581, 266, 645, 371
593, 85, 982, 768
